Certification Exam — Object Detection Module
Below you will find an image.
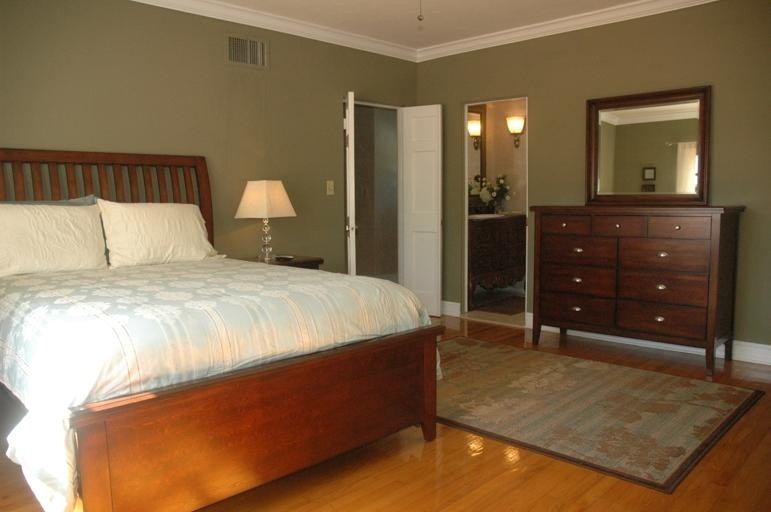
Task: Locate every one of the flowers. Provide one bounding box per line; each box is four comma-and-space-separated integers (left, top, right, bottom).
480, 174, 517, 212
468, 174, 482, 196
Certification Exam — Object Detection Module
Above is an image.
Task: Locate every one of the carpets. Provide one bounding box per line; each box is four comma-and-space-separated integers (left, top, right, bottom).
466, 294, 526, 326
435, 332, 767, 495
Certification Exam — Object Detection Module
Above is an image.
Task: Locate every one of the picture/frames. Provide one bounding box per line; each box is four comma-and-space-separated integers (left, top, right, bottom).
643, 166, 657, 181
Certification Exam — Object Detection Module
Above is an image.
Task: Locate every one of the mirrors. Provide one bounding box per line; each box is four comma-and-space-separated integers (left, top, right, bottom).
585, 84, 713, 205
467, 105, 487, 207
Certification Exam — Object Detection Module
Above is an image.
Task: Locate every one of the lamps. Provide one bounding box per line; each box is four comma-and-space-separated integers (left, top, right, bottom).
232, 178, 299, 262
505, 114, 526, 149
468, 119, 482, 150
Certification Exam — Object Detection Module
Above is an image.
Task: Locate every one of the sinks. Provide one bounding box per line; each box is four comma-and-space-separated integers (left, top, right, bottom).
468, 212, 507, 221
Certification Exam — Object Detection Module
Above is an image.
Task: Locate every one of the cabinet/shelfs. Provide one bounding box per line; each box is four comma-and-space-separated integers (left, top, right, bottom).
468, 215, 527, 279
528, 202, 747, 378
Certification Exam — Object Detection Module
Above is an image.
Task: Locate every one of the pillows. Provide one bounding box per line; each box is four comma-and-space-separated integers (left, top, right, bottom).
0, 193, 227, 280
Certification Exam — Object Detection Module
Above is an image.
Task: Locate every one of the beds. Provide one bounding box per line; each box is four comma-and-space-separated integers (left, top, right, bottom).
1, 145, 447, 511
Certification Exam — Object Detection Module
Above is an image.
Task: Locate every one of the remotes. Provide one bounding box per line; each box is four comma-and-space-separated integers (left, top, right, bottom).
275, 255, 294, 259
